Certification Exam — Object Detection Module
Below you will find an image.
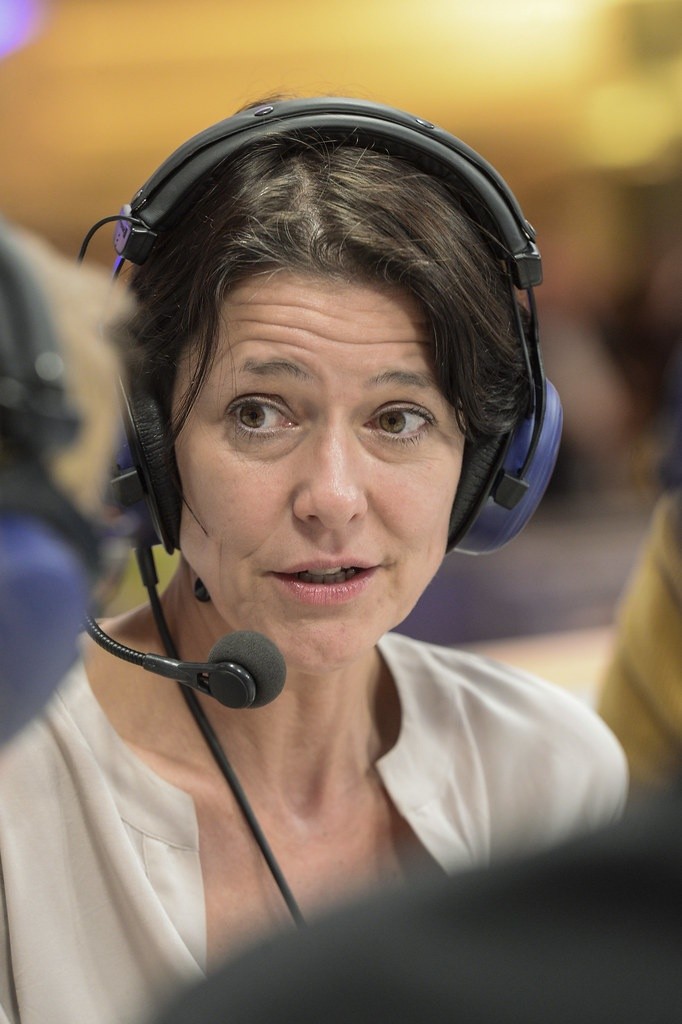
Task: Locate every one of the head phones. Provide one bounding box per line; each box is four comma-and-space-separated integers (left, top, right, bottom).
76, 99, 564, 710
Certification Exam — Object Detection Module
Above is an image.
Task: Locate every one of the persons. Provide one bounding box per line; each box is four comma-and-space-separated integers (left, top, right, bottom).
0, 82, 628, 1024
591, 428, 682, 799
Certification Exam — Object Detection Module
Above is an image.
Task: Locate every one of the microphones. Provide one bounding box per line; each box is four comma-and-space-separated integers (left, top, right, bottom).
142, 631, 285, 710
